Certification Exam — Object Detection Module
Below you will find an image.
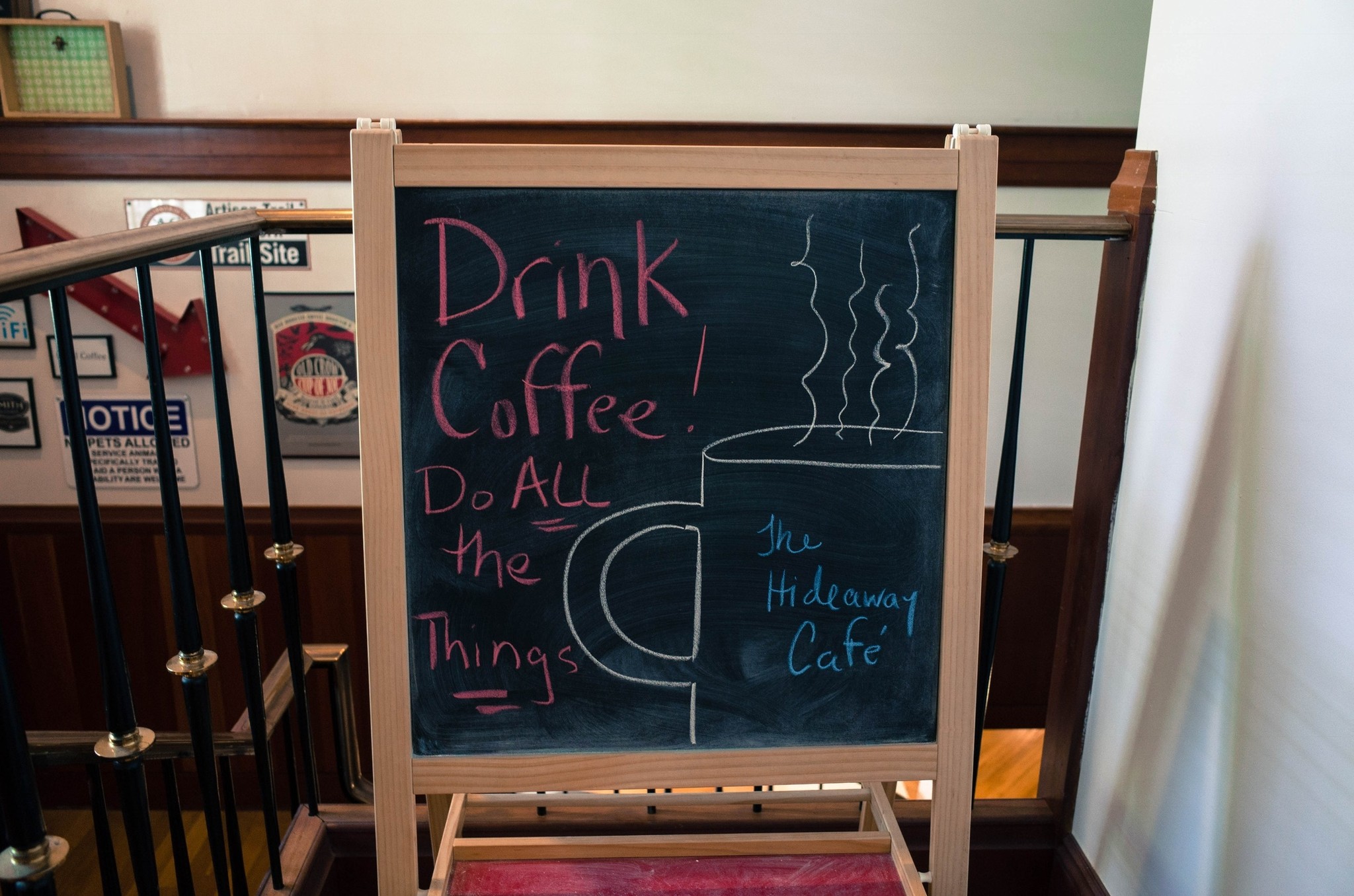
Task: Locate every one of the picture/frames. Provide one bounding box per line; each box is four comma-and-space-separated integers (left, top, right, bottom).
261, 292, 361, 459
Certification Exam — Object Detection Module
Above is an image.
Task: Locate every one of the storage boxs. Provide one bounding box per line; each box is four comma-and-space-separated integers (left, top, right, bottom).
0, 20, 132, 123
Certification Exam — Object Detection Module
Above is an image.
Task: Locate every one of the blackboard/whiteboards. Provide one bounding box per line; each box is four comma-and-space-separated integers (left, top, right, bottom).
349, 117, 999, 793
